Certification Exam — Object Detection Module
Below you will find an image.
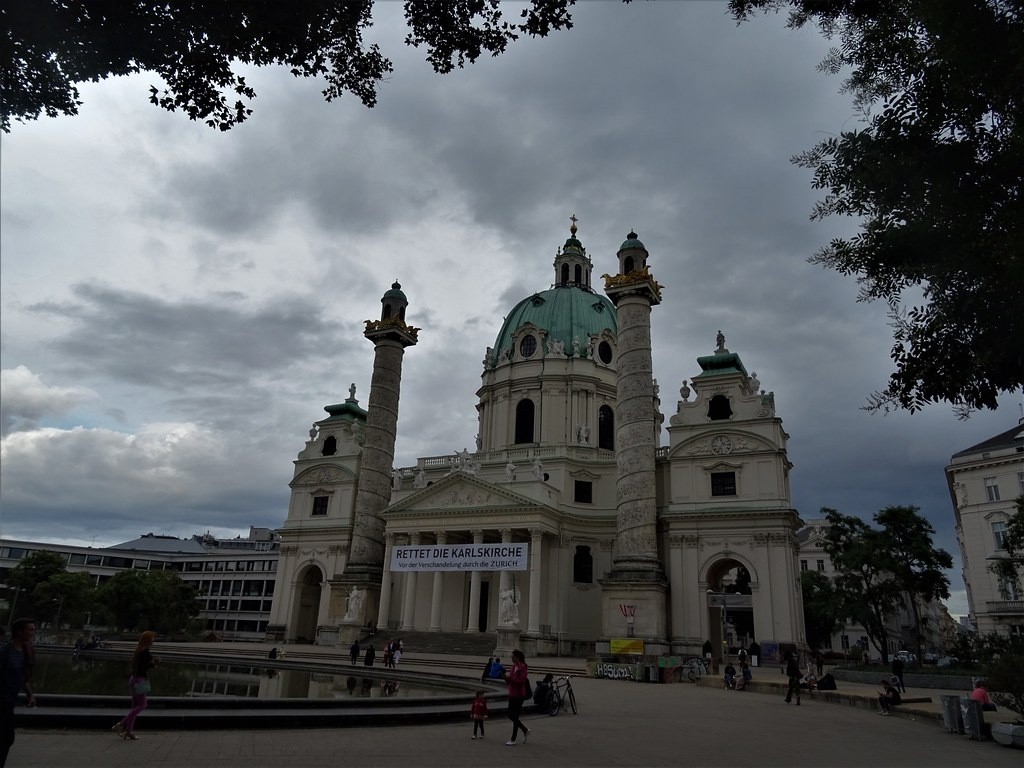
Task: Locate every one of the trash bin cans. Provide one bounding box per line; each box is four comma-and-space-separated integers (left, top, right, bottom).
940, 695, 964, 733
960, 698, 984, 741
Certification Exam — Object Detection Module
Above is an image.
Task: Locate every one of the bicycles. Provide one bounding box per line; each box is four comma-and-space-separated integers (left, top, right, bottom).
545, 674, 578, 717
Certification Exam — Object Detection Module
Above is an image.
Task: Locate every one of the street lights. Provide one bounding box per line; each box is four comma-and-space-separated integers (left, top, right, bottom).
7, 584, 26, 627
706, 586, 742, 666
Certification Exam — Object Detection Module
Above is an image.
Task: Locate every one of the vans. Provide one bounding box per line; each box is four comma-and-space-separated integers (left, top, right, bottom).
897, 650, 910, 661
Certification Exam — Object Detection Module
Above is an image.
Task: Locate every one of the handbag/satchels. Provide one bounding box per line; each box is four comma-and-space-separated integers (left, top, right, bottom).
524, 679, 532, 699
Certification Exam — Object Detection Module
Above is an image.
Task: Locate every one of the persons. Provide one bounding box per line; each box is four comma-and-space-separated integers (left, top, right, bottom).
111, 631, 161, 740
505, 460, 519, 480
498, 346, 508, 359
268, 647, 277, 659
482, 658, 506, 679
390, 467, 403, 488
533, 673, 553, 715
368, 619, 379, 638
350, 637, 406, 668
784, 651, 824, 706
342, 586, 366, 623
579, 424, 587, 444
470, 690, 488, 739
724, 646, 752, 690
475, 434, 481, 450
971, 678, 998, 712
455, 448, 469, 470
73, 634, 96, 652
553, 335, 593, 356
0, 618, 36, 768
529, 457, 544, 479
877, 658, 906, 715
347, 677, 400, 698
504, 649, 532, 746
716, 330, 725, 349
414, 468, 427, 487
500, 586, 521, 625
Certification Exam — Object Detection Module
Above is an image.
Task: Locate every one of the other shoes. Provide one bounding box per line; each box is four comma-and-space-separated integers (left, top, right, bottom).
472, 736, 477, 739
480, 735, 485, 739
796, 701, 801, 705
878, 711, 887, 715
523, 729, 532, 744
725, 687, 727, 689
784, 699, 790, 703
730, 684, 732, 687
883, 712, 892, 716
506, 740, 517, 746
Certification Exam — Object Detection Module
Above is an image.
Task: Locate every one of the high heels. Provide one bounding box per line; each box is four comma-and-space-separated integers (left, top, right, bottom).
124, 732, 140, 741
111, 726, 124, 737
